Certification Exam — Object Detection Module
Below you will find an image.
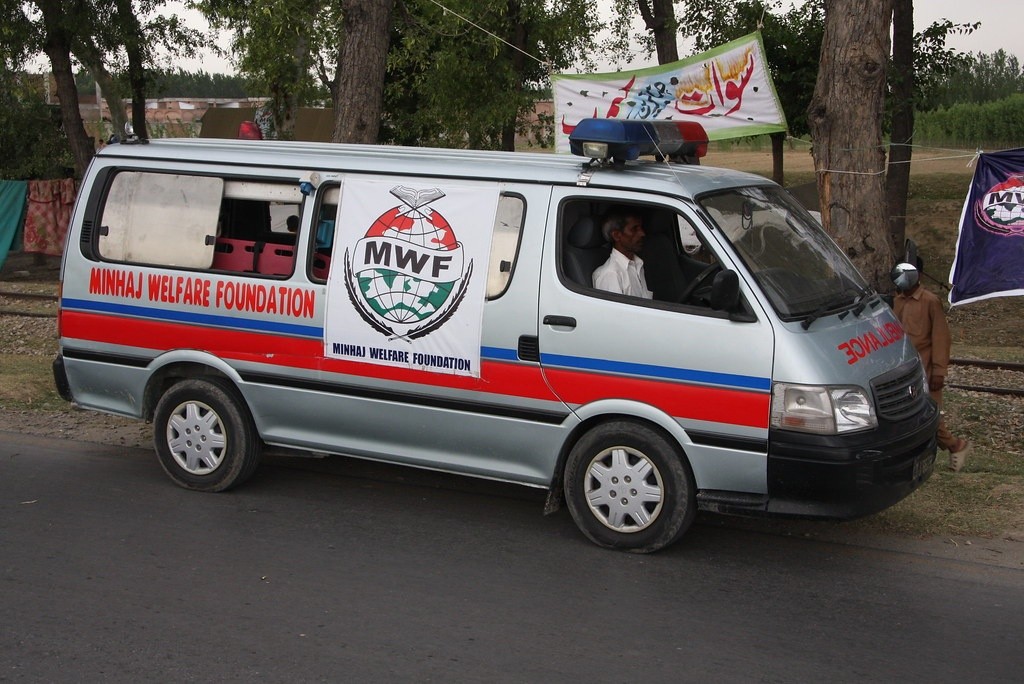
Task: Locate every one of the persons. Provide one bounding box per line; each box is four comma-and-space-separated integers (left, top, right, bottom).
591, 207, 654, 301
892, 254, 973, 474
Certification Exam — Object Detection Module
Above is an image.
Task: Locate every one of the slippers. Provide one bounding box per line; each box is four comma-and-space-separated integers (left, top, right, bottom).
948, 440, 972, 473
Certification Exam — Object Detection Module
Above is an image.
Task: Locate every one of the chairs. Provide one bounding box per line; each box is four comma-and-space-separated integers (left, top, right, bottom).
562, 213, 610, 288
641, 232, 689, 301
216, 240, 331, 280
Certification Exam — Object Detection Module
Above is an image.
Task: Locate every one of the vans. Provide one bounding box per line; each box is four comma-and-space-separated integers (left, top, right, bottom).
51, 115, 941, 556
